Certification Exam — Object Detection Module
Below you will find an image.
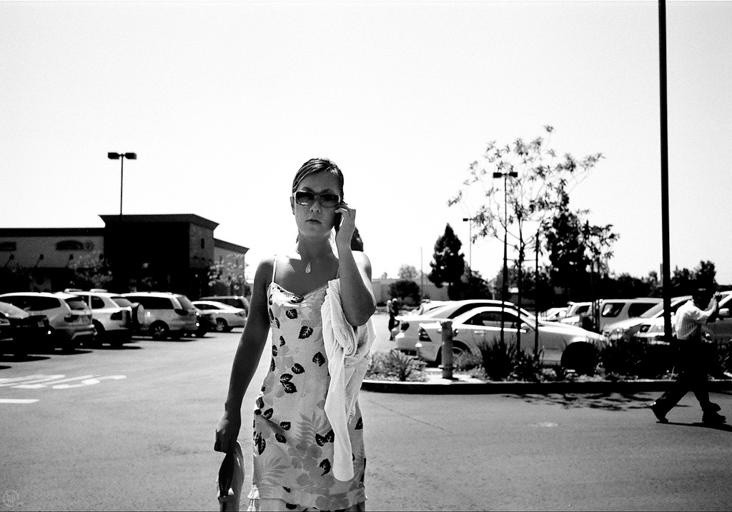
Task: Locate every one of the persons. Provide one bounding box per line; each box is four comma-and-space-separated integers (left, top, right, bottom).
214, 156, 378, 511
648, 289, 727, 425
386, 298, 400, 341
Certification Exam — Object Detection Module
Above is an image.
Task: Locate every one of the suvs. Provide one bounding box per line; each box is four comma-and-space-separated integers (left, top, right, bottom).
0, 288, 201, 355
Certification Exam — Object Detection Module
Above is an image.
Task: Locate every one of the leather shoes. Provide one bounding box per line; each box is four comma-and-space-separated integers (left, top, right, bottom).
651, 401, 669, 424
703, 409, 725, 424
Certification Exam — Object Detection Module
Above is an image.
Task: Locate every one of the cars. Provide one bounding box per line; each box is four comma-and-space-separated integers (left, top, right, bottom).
191, 296, 249, 337
392, 298, 611, 374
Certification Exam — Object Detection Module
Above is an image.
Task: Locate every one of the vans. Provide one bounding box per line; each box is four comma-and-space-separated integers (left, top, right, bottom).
543, 290, 731, 351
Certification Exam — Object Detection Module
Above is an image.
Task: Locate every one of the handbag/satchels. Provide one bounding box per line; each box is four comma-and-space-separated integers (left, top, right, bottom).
217, 440, 246, 511
674, 339, 709, 376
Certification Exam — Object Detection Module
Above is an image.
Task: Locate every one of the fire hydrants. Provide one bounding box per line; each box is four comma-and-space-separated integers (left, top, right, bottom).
437, 318, 458, 379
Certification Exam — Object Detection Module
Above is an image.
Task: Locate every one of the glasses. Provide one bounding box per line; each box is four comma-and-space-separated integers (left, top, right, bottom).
295, 191, 340, 207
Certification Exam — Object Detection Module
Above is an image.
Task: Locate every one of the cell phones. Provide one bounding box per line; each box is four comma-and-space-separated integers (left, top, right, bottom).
335, 200, 345, 231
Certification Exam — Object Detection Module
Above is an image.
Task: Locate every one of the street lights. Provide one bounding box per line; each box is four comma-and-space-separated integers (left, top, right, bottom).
493, 171, 518, 340
108, 152, 137, 216
463, 217, 476, 269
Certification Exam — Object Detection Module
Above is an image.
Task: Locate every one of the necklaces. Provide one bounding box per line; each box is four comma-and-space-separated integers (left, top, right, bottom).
300, 241, 335, 274
